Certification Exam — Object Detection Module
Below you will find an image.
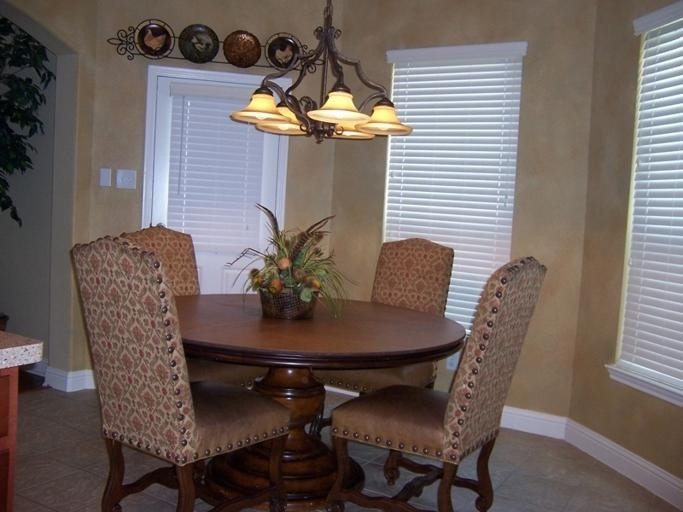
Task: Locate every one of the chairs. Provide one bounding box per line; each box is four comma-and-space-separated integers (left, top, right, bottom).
310, 238, 456, 477
333, 252, 548, 506
70, 236, 291, 508
118, 223, 277, 489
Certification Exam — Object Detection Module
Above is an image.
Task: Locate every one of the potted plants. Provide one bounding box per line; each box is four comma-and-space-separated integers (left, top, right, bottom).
228, 203, 359, 321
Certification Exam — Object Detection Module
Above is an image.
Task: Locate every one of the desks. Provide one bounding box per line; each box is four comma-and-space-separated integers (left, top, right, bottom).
167, 293, 466, 511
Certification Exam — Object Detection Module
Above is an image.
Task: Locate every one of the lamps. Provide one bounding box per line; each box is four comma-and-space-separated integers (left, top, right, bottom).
230, 0, 412, 141
